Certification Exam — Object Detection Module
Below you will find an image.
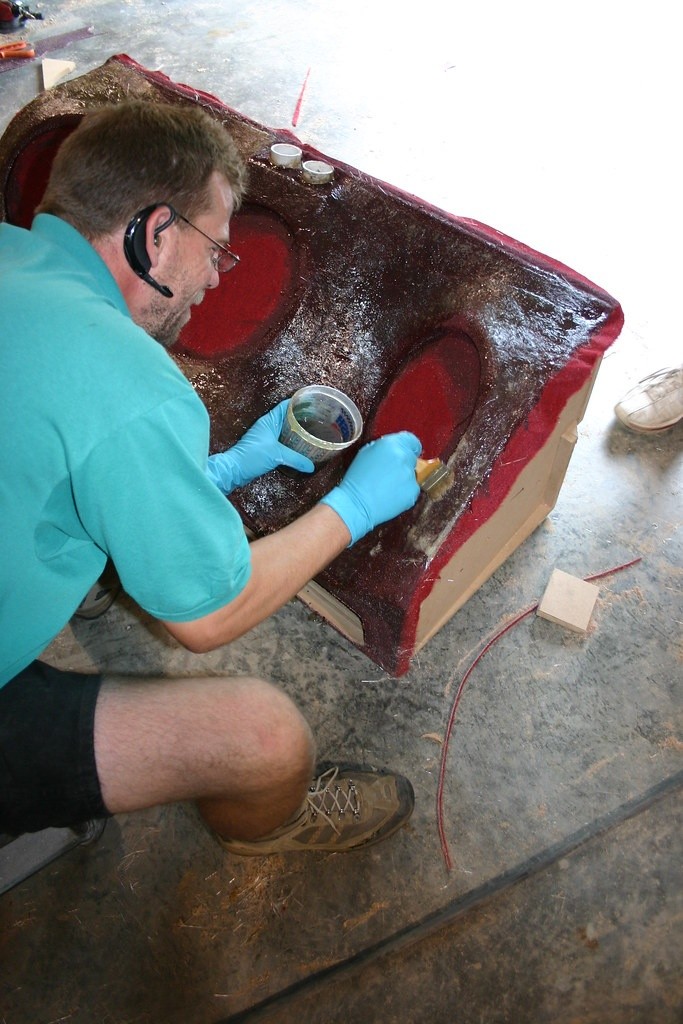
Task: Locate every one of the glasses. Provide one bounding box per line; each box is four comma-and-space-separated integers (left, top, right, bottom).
173, 211, 241, 273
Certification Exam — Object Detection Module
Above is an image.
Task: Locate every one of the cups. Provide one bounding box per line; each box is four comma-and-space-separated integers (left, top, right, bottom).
273, 385, 364, 480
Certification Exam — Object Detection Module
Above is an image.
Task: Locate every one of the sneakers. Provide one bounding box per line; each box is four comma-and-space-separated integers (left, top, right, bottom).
71, 556, 121, 617
614, 365, 683, 433
214, 761, 413, 855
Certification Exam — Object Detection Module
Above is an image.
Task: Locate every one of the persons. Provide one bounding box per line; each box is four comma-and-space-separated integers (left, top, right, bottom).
0, 100, 423, 856
614, 363, 683, 436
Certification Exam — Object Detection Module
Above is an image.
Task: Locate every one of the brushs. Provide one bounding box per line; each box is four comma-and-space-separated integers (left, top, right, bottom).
414, 458, 456, 502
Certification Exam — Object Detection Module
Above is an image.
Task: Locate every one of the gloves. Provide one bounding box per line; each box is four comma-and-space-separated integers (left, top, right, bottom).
201, 396, 314, 497
319, 430, 424, 548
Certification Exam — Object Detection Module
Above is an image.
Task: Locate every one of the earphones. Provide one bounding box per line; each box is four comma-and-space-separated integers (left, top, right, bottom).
152, 231, 161, 247
